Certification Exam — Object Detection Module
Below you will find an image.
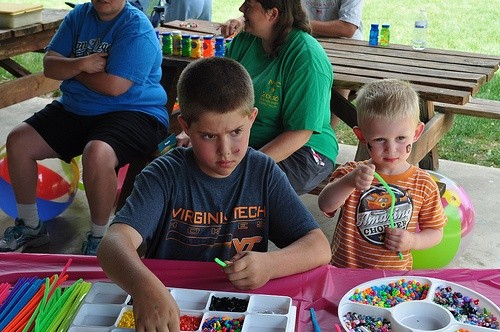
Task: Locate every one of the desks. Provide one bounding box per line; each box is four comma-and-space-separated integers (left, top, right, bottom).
156, 18, 500, 171
0, 9, 73, 78
0, 253, 500, 332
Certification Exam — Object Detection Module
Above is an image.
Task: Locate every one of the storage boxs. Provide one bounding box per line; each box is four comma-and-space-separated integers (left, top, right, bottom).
0, 5, 44, 28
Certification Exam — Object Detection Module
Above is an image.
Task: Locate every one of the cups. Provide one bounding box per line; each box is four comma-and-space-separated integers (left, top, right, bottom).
151, 7, 165, 28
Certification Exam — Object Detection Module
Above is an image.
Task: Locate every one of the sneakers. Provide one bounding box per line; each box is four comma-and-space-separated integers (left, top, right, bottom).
81, 232, 104, 257
0, 216, 51, 254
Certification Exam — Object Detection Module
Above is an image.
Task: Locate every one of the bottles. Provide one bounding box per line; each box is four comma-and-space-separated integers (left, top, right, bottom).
369, 24, 379, 45
191, 35, 201, 57
156, 30, 172, 53
413, 10, 428, 50
215, 37, 232, 57
172, 31, 181, 55
182, 34, 190, 56
380, 24, 390, 46
203, 36, 214, 58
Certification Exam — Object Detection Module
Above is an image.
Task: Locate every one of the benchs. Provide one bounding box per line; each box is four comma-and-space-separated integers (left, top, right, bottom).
434, 98, 500, 119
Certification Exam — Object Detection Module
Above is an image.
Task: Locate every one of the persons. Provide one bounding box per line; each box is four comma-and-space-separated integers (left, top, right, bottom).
318, 80, 448, 269
222, 0, 364, 129
163, 0, 212, 23
97, 55, 331, 332
176, 0, 339, 196
0, 0, 170, 256
